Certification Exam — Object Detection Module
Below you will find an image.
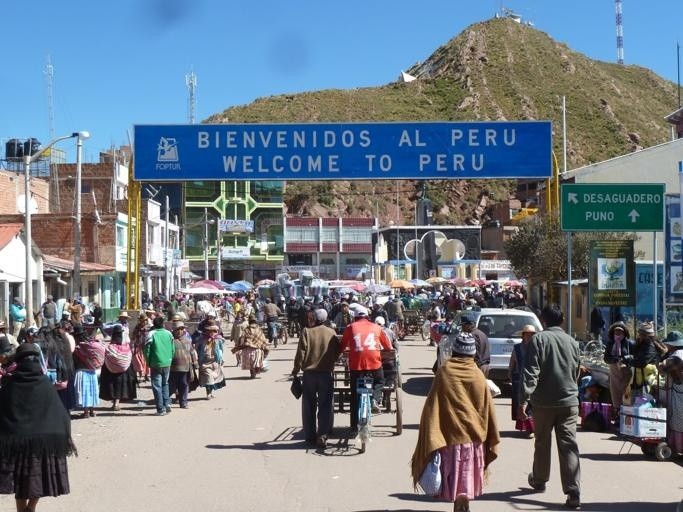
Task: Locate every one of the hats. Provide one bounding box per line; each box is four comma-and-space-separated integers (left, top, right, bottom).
353, 308, 367, 318
0, 336, 15, 355
661, 330, 683, 348
155, 292, 183, 300
460, 312, 476, 325
62, 311, 258, 335
452, 321, 477, 355
276, 292, 400, 304
638, 320, 656, 337
513, 325, 540, 336
374, 315, 387, 325
15, 342, 42, 360
14, 296, 21, 302
47, 294, 53, 300
314, 308, 328, 322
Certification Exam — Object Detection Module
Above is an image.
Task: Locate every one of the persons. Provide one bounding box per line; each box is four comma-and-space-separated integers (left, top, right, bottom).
603, 321, 632, 420
289, 308, 338, 454
287, 287, 407, 340
0, 295, 281, 413
626, 322, 660, 400
0, 340, 78, 512
588, 306, 605, 344
426, 331, 484, 511
335, 301, 392, 432
658, 329, 683, 461
399, 282, 525, 317
520, 300, 585, 511
507, 323, 536, 439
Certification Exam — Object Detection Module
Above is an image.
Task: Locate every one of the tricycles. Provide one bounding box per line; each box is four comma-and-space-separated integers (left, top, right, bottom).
231, 307, 432, 344
326, 341, 404, 455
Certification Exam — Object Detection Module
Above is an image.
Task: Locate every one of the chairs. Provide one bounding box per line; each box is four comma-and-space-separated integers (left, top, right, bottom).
479, 324, 518, 338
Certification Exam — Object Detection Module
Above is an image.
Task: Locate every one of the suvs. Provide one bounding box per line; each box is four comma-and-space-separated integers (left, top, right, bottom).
430, 306, 546, 390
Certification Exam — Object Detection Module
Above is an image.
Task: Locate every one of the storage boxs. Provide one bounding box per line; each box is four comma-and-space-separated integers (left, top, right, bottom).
620, 406, 667, 437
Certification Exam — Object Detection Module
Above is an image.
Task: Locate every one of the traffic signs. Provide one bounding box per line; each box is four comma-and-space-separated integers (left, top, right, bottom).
560, 183, 665, 233
130, 120, 554, 182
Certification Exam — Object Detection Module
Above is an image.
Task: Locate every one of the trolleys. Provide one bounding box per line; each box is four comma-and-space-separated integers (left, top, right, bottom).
612, 367, 674, 460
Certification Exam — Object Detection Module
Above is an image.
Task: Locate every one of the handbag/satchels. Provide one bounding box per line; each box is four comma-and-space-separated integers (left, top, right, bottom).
416, 451, 443, 498
290, 375, 302, 399
630, 381, 652, 398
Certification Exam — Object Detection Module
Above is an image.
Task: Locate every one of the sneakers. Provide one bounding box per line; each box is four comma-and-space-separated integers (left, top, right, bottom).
565, 493, 581, 508
156, 404, 190, 416
82, 412, 97, 418
527, 472, 546, 493
111, 404, 121, 411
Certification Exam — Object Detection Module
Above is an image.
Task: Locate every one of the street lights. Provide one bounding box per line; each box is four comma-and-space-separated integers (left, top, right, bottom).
181, 220, 216, 286
21, 130, 92, 330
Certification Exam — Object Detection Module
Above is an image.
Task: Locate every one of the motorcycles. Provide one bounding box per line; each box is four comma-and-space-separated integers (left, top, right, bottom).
241, 346, 260, 378
